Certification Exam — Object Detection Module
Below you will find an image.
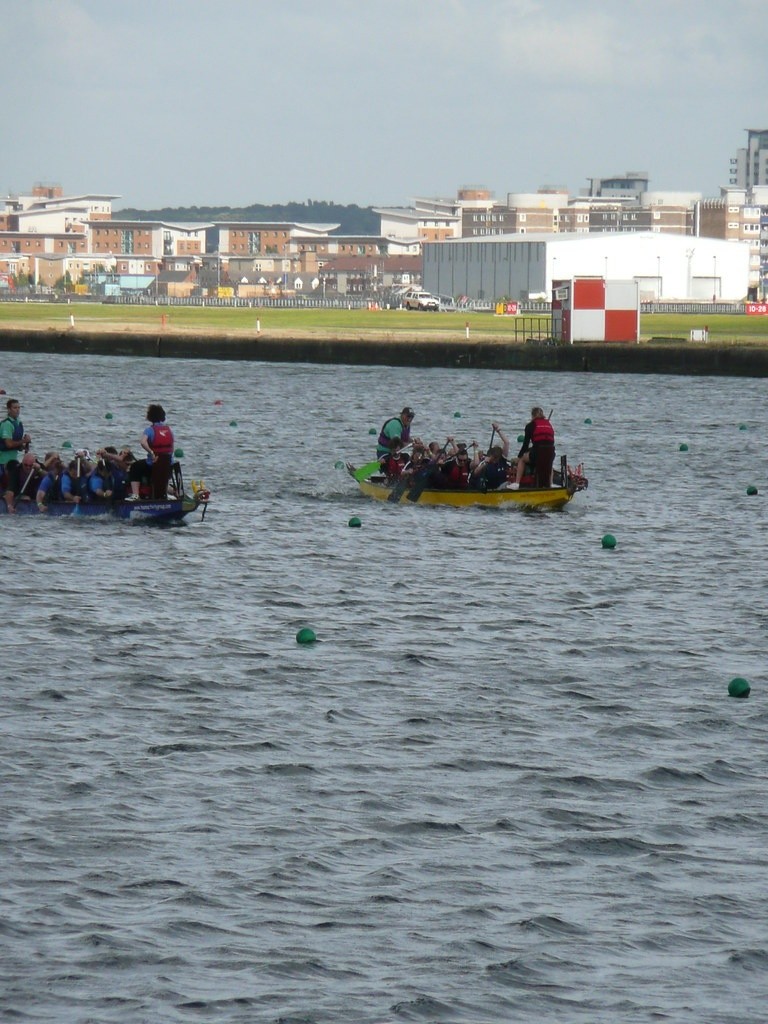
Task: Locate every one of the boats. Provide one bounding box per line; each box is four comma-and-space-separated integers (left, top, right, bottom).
344, 455, 587, 511
0, 461, 213, 523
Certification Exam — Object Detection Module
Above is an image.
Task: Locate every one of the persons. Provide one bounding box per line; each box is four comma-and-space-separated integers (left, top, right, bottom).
0, 447, 133, 513
507, 408, 561, 490
125, 404, 174, 501
378, 408, 420, 476
0, 399, 31, 478
380, 423, 512, 490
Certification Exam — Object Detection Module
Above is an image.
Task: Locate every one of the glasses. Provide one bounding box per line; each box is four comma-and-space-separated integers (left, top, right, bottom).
458, 458, 467, 462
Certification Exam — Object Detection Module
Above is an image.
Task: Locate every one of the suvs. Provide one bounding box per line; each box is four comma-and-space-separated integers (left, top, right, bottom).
403, 291, 440, 312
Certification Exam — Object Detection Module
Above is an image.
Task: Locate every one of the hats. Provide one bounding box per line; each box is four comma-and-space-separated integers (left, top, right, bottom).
402, 407, 415, 416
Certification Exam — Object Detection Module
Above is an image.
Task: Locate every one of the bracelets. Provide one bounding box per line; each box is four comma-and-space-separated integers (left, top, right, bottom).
7, 505, 11, 508
150, 451, 153, 454
104, 493, 106, 497
497, 429, 500, 432
428, 453, 432, 456
38, 503, 42, 506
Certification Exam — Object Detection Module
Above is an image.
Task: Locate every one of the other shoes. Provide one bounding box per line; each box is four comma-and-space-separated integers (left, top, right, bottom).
506, 483, 520, 491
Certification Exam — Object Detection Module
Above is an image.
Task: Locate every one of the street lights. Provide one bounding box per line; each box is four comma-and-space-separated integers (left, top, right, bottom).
552, 257, 556, 279
656, 255, 661, 311
713, 256, 717, 312
605, 256, 608, 280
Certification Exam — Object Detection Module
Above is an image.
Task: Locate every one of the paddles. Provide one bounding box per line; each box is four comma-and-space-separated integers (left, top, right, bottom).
12, 450, 191, 516
352, 409, 555, 504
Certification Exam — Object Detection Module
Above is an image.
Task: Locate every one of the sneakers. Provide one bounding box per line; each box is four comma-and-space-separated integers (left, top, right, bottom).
124, 494, 140, 501
